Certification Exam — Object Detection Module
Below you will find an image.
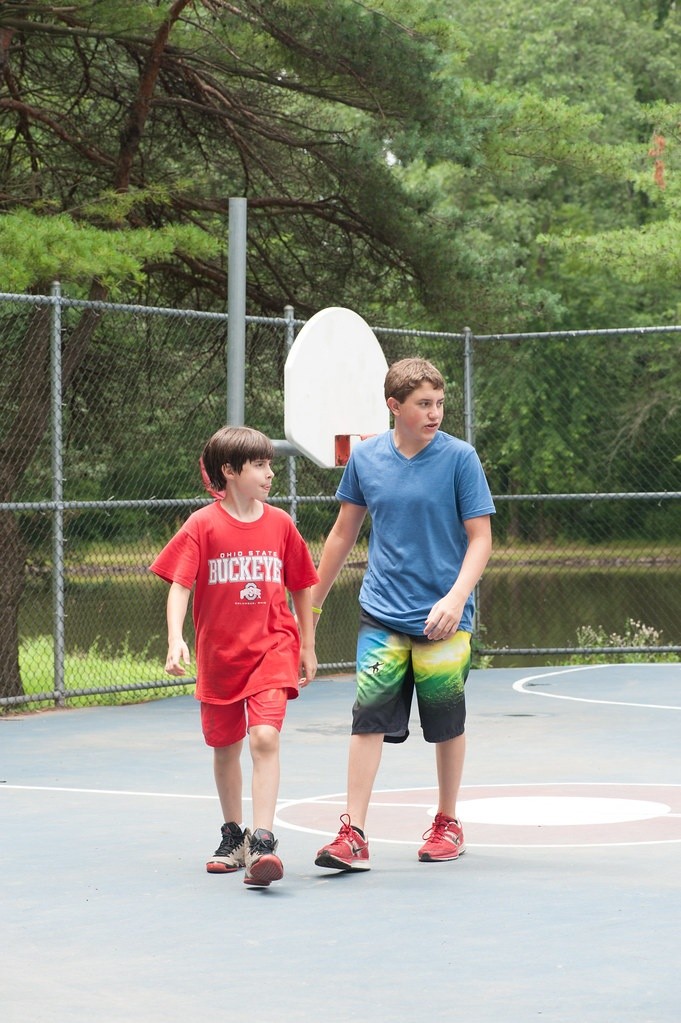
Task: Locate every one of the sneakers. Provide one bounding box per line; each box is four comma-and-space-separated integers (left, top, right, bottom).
206, 820, 250, 872
316, 814, 371, 871
418, 813, 465, 862
244, 828, 284, 886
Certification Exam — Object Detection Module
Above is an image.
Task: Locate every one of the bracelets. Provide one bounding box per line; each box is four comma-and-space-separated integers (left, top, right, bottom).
312, 606, 322, 614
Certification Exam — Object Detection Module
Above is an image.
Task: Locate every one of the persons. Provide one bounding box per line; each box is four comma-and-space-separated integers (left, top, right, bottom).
149, 426, 317, 886
312, 358, 497, 872
